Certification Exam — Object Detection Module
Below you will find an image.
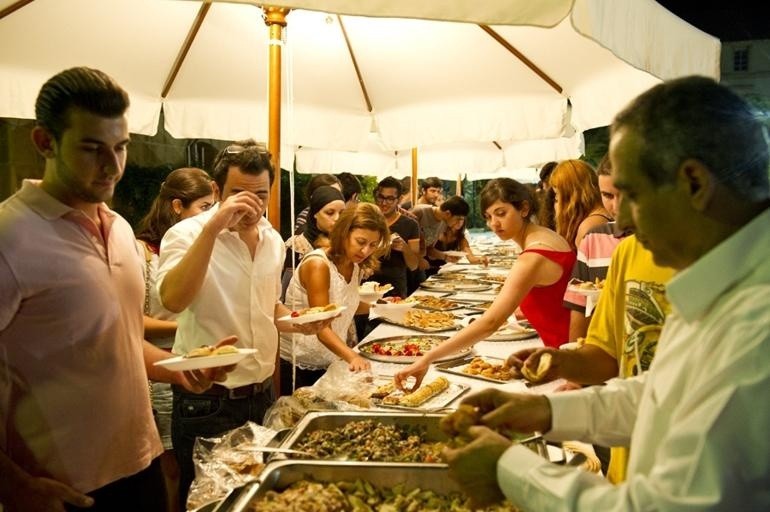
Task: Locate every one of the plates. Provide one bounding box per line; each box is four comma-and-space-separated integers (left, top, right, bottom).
278, 304, 347, 324
384, 310, 460, 331
358, 334, 471, 363
379, 379, 470, 412
421, 280, 492, 291
444, 251, 468, 256
359, 282, 391, 295
462, 313, 538, 340
447, 293, 499, 302
416, 297, 465, 311
470, 301, 493, 311
154, 347, 258, 370
435, 354, 507, 384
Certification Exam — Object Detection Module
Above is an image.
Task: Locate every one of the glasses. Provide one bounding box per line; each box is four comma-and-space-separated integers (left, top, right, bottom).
225, 144, 268, 156
375, 194, 399, 204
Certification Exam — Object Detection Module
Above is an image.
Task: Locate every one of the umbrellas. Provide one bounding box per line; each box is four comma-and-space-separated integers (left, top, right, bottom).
0, 0, 723, 232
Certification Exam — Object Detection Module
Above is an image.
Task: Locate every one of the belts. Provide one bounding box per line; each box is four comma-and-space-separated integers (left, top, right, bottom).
210, 379, 270, 402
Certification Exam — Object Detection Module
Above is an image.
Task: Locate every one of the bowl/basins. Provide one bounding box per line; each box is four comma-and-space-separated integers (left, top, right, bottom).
267, 408, 552, 462
213, 463, 519, 512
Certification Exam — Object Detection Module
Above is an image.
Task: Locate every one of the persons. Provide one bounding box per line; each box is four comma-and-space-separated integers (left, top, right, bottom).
134, 166, 219, 354
428, 215, 483, 265
408, 196, 469, 271
439, 73, 770, 512
564, 150, 620, 390
157, 138, 343, 512
280, 184, 347, 304
2, 65, 243, 512
398, 175, 419, 209
417, 176, 443, 207
505, 229, 678, 489
353, 176, 421, 343
393, 176, 576, 399
278, 200, 393, 398
334, 172, 363, 202
293, 172, 344, 234
